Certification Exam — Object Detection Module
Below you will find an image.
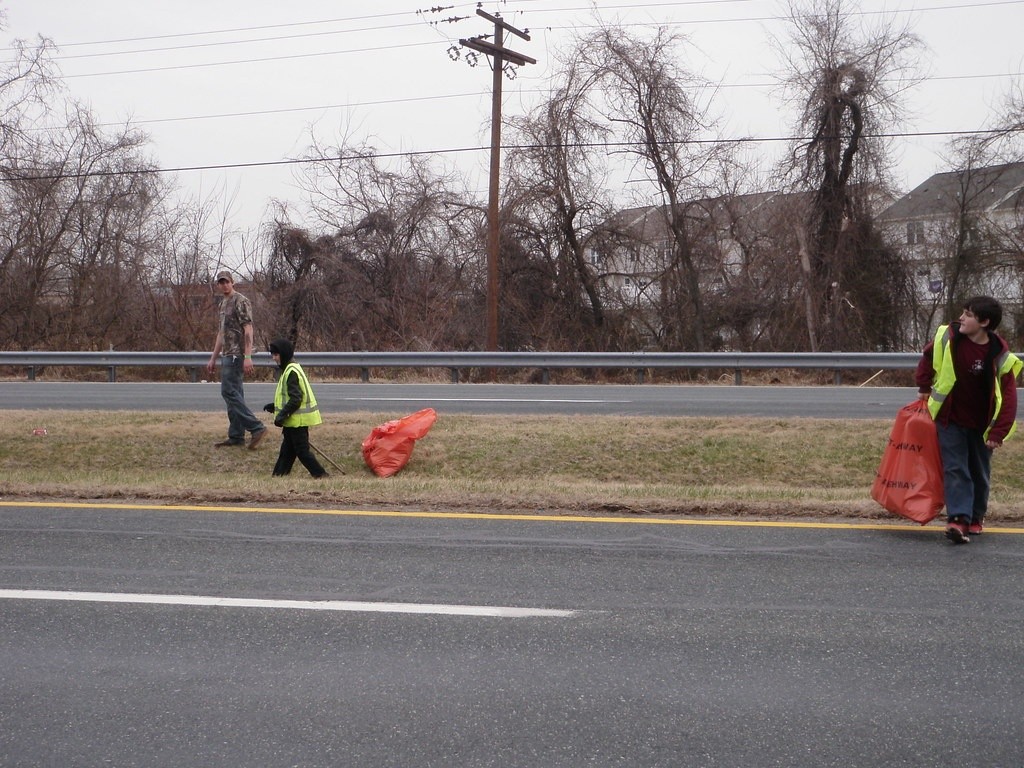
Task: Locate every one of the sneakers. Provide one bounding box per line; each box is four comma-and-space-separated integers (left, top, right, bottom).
968, 515, 986, 534
215, 439, 245, 448
248, 427, 266, 450
945, 516, 970, 544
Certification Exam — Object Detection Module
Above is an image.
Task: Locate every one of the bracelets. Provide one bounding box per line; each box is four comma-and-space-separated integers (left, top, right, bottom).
244, 355, 252, 358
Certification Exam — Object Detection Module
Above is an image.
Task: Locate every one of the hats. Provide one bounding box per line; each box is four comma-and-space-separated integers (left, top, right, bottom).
217, 271, 232, 283
270, 345, 279, 354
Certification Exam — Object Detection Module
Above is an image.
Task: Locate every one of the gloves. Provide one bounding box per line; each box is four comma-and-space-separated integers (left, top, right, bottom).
275, 416, 285, 427
263, 403, 275, 414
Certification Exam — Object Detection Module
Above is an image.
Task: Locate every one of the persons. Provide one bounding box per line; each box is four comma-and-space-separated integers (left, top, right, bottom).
207, 270, 269, 451
263, 339, 330, 479
915, 295, 1023, 544
